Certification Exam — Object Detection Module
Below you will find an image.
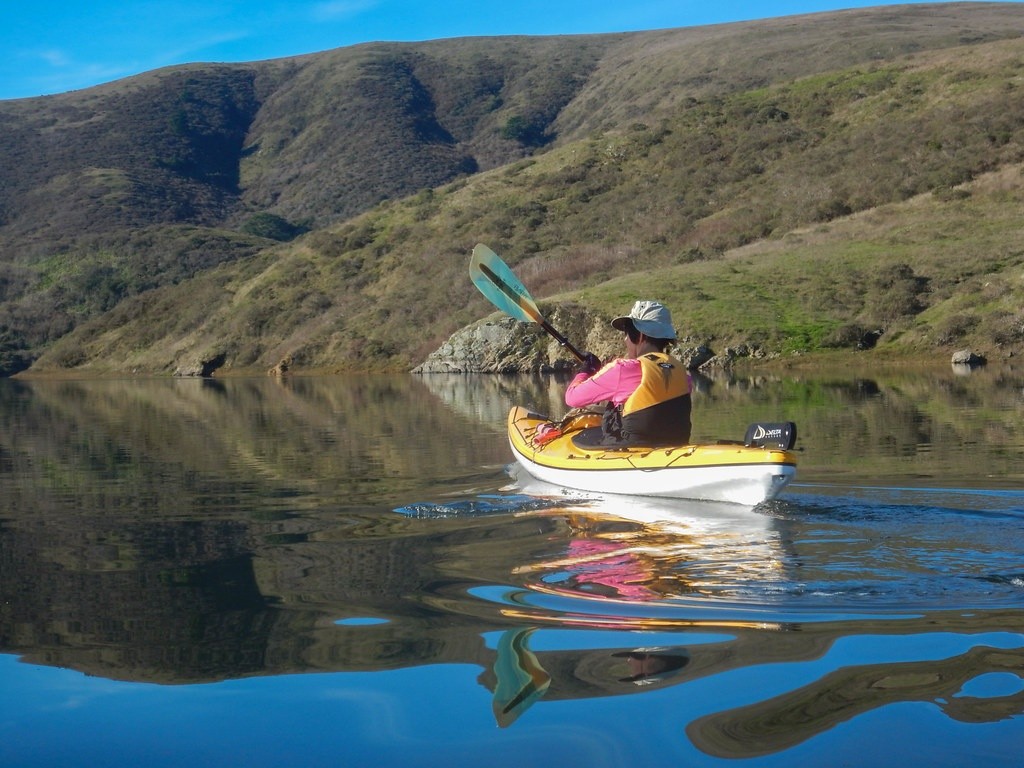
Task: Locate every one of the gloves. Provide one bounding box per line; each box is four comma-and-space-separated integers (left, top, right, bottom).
578, 351, 602, 375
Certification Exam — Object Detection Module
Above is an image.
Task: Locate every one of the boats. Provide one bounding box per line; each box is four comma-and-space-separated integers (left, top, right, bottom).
508, 405, 805, 506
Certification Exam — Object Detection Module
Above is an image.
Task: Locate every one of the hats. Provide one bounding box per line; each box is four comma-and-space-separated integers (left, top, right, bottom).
610, 300, 677, 340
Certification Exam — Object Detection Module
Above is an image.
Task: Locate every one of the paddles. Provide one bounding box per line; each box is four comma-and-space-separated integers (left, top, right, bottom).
467, 242, 586, 363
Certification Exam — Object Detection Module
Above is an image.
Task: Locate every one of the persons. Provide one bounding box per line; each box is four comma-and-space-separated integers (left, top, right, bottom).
565, 300, 692, 448
